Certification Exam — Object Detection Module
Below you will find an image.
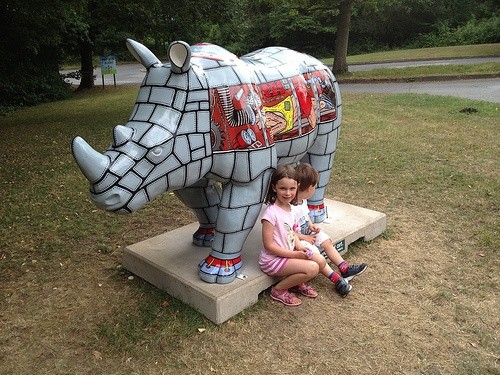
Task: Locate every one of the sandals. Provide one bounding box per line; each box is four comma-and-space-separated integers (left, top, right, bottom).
270, 286, 301, 306
291, 284, 317, 297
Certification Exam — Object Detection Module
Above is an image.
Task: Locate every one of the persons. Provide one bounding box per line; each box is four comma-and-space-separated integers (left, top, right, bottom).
257, 165, 326, 306
289, 163, 369, 298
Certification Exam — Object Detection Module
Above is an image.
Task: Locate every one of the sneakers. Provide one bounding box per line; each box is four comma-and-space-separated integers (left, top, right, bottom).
340, 263, 367, 280
335, 278, 352, 298
